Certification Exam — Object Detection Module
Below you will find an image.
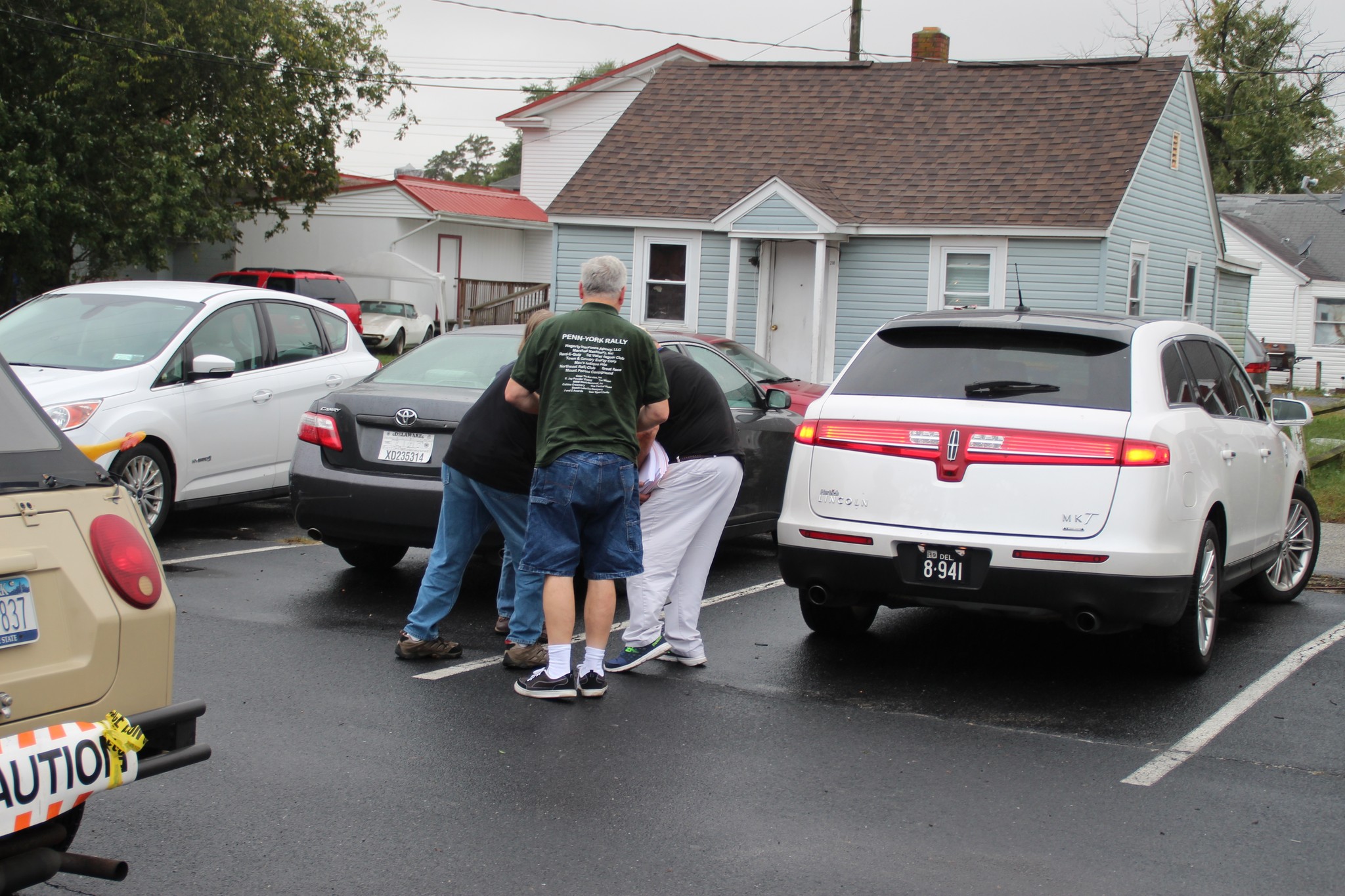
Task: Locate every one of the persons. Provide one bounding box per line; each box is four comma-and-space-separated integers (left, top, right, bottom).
505, 256, 669, 697
602, 333, 746, 673
395, 310, 550, 670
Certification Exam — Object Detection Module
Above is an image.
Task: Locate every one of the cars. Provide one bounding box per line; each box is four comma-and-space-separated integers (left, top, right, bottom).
0, 278, 384, 542
0, 354, 216, 896
289, 323, 805, 599
652, 330, 829, 417
1244, 327, 1273, 404
359, 300, 436, 356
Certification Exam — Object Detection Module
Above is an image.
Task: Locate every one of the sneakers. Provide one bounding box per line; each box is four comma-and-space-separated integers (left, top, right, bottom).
514, 668, 577, 699
577, 668, 608, 697
604, 637, 673, 672
504, 640, 550, 668
659, 650, 709, 666
395, 629, 463, 659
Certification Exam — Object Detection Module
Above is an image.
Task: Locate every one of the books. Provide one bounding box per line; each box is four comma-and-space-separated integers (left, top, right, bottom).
638, 438, 670, 495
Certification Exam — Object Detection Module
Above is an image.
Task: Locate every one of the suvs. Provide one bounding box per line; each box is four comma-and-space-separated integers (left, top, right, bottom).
204, 266, 363, 340
776, 305, 1322, 678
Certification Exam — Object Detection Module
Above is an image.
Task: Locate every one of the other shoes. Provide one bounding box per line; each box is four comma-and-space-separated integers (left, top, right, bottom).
496, 613, 512, 633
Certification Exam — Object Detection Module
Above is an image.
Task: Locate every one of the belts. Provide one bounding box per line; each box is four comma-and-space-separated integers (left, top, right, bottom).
668, 452, 717, 464
